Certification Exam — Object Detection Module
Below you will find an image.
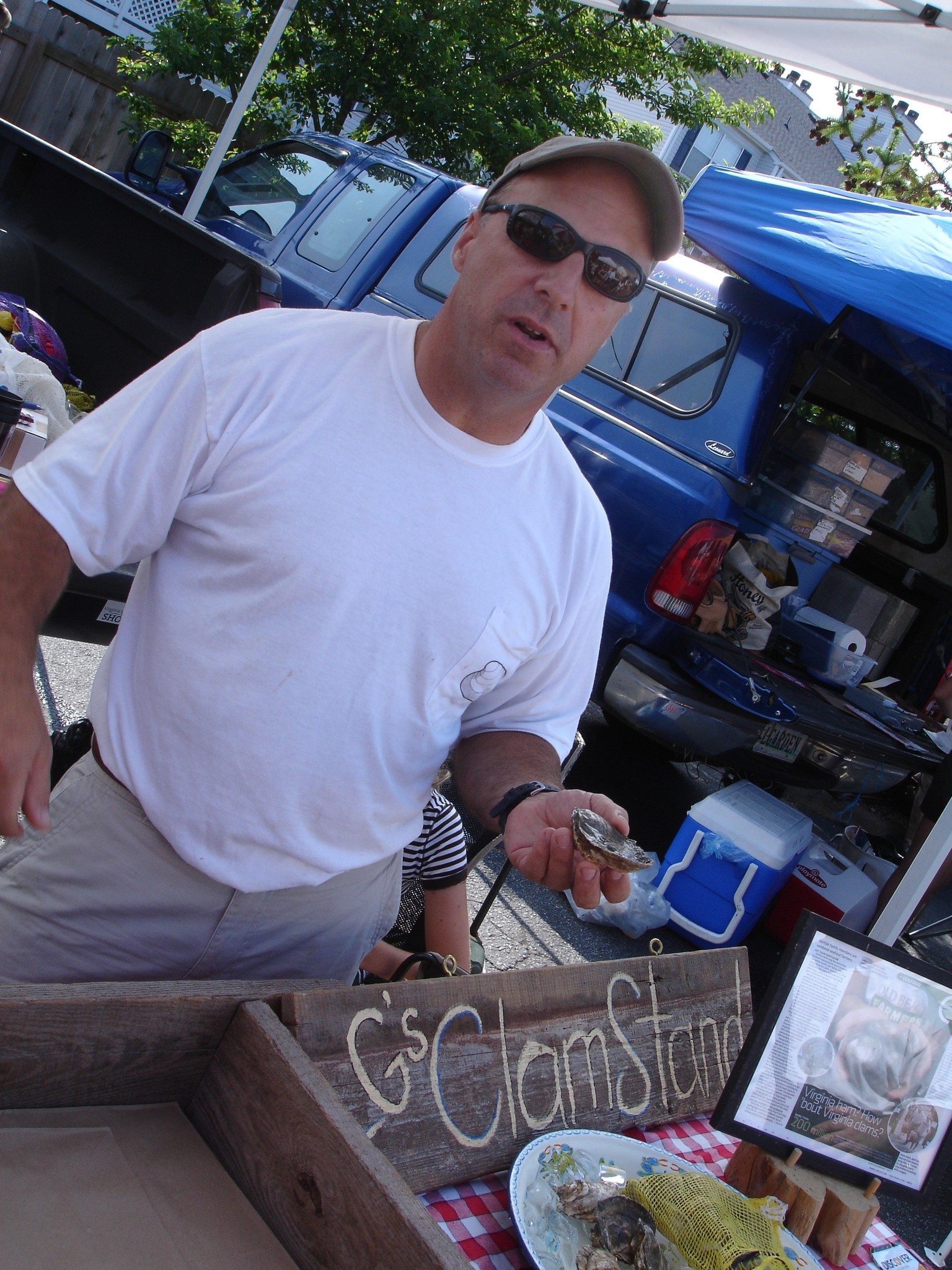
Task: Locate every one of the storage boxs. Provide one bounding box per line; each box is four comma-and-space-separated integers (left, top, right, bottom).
648, 778, 879, 948
0, 407, 48, 476
776, 614, 879, 687
731, 418, 907, 604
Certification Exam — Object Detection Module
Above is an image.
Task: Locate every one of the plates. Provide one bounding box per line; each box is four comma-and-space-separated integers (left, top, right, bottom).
507, 1129, 826, 1270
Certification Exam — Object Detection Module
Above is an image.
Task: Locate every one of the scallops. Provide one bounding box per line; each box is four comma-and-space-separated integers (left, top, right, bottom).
571, 805, 655, 873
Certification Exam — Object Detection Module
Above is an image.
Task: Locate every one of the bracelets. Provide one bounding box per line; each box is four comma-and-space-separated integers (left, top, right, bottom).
489, 781, 561, 835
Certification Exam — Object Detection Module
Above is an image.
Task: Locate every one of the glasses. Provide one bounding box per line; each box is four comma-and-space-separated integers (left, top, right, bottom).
481, 202, 648, 303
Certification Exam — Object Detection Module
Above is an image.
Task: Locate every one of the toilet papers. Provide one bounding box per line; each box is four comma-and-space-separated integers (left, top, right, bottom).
793, 606, 867, 656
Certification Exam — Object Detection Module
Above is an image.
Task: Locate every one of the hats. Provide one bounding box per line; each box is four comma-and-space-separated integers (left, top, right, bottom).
475, 135, 686, 262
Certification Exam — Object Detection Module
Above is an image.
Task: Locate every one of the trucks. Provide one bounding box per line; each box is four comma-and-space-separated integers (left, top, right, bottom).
104, 130, 952, 798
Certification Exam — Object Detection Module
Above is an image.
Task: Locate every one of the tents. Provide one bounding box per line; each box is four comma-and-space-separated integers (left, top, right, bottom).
678, 164, 951, 362
183, 0, 952, 232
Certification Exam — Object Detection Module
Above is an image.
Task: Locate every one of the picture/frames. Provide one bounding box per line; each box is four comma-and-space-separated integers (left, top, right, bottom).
709, 907, 952, 1209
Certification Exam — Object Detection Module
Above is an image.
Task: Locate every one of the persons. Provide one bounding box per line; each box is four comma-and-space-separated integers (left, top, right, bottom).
0, 131, 685, 1015
352, 788, 471, 987
